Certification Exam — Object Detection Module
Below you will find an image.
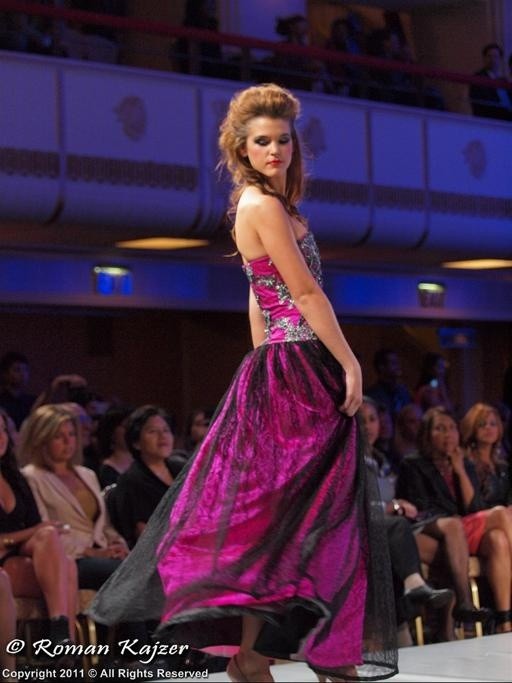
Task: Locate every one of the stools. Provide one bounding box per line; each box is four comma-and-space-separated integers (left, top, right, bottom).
10, 586, 103, 675
410, 553, 483, 648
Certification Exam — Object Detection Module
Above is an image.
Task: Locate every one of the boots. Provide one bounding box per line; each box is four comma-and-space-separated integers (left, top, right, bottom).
39, 615, 79, 655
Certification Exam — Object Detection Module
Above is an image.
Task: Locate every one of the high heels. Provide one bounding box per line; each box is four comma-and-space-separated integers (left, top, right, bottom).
225, 652, 360, 681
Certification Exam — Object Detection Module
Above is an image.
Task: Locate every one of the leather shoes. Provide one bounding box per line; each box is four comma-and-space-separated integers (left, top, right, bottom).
401, 581, 510, 631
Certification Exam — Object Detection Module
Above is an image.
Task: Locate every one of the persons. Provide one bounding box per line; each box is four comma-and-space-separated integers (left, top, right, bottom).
80, 78, 402, 683
